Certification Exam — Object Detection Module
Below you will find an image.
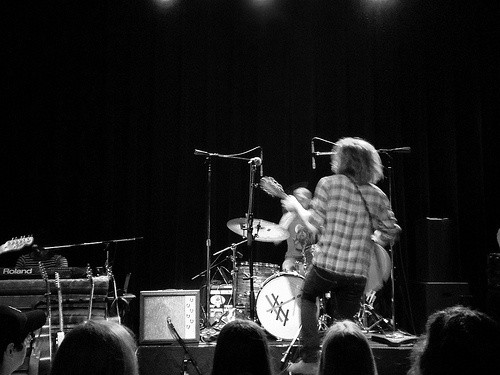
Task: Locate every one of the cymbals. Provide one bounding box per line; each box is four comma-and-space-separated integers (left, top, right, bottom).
228, 216, 290, 242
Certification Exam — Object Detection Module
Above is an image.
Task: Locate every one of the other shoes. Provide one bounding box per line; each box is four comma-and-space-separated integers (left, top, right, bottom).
288, 359, 319, 375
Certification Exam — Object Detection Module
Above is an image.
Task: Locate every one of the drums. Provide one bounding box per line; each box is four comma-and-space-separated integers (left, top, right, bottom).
259, 270, 319, 339
234, 262, 281, 314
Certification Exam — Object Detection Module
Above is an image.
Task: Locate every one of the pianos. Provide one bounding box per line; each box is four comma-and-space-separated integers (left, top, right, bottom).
0, 264, 122, 323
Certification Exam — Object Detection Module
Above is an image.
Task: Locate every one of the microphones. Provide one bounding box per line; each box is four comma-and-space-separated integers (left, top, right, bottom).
167, 317, 171, 325
260, 148, 264, 176
312, 139, 316, 169
32, 244, 40, 254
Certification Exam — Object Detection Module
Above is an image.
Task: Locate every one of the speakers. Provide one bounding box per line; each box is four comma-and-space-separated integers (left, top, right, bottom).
140, 289, 200, 344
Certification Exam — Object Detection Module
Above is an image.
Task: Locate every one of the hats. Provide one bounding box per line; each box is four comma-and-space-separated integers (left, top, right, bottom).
0, 304, 46, 342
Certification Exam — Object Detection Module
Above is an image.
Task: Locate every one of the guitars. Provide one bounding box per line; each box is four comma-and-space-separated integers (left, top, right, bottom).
260, 174, 382, 295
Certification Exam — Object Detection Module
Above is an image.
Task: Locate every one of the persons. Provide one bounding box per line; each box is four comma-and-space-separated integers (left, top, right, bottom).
0, 305, 46, 375
407, 305, 500, 375
273, 181, 320, 279
50, 320, 139, 374
281, 137, 403, 375
15, 230, 69, 268
319, 319, 378, 374
210, 319, 272, 375
